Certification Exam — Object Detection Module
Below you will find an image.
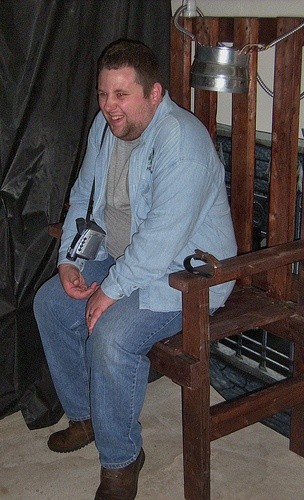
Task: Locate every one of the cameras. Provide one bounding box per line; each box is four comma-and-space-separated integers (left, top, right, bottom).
67, 218, 106, 260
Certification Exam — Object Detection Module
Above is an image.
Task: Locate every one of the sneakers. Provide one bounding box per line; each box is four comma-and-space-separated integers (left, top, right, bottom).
94, 447, 145, 500
47, 420, 95, 453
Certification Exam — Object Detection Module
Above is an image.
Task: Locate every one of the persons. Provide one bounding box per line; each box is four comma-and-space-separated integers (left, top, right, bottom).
32, 39, 238, 500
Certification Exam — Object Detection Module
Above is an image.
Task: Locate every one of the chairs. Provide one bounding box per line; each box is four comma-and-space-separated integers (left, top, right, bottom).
47, 16, 304, 500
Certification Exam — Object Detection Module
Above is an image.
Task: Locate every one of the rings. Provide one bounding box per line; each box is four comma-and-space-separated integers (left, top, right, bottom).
87, 313, 94, 318
